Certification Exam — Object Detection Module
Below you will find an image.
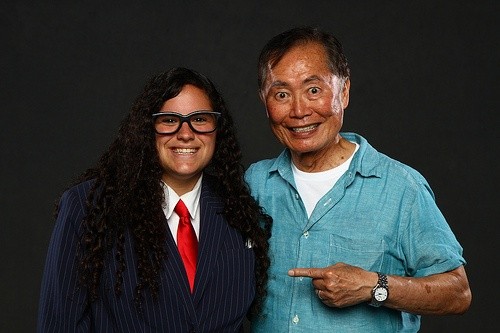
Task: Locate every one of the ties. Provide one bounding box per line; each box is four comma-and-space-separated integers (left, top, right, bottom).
174, 199, 199, 293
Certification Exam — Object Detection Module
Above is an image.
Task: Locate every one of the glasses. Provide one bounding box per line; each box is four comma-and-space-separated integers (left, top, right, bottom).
151, 110, 222, 135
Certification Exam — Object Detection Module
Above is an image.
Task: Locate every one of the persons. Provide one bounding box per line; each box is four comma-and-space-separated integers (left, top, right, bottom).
39, 66, 277, 333
238, 26, 474, 333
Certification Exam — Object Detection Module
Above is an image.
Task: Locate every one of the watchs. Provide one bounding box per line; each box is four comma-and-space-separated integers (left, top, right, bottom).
369, 271, 391, 309
